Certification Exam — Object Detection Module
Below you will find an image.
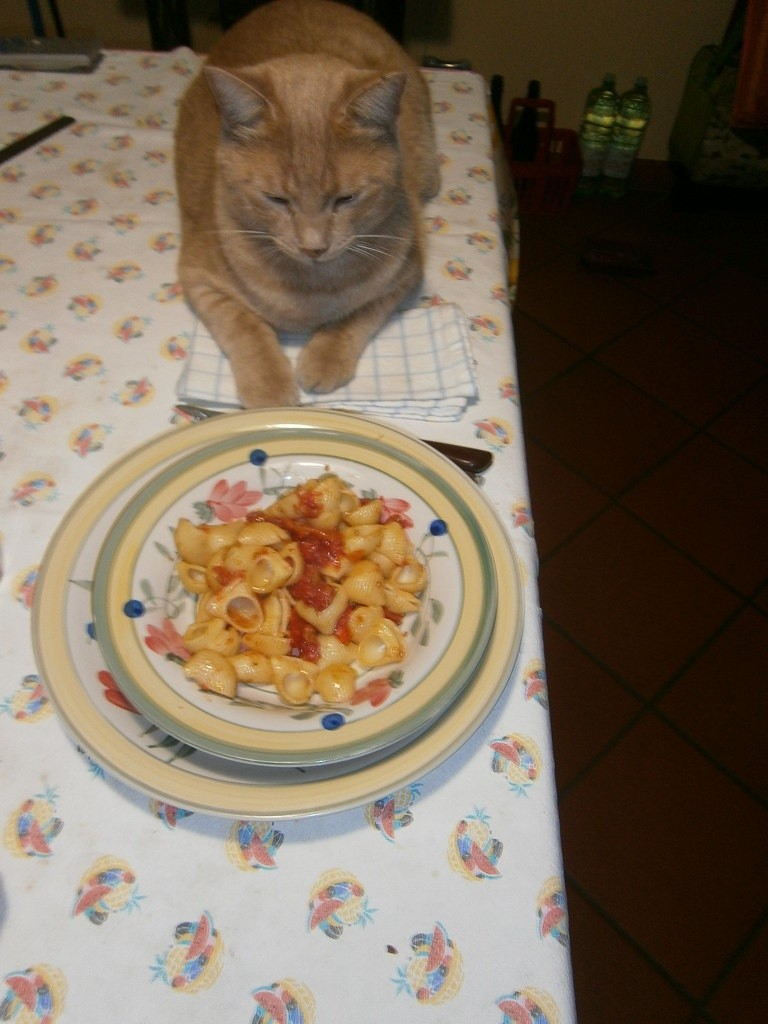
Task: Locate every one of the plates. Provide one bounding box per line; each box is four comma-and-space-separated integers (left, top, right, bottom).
29, 407, 527, 822
90, 429, 498, 767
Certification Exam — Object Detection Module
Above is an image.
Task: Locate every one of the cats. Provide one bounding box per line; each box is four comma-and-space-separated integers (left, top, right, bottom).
171, 0, 442, 412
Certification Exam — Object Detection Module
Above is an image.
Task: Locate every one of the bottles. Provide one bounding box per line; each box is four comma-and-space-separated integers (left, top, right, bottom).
578, 72, 652, 179
488, 73, 543, 165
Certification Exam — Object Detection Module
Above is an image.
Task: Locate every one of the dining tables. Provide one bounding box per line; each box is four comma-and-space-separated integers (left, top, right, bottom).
0, 48, 578, 1023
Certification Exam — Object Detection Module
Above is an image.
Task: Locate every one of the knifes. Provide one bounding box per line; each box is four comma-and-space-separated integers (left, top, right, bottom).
176, 403, 493, 475
